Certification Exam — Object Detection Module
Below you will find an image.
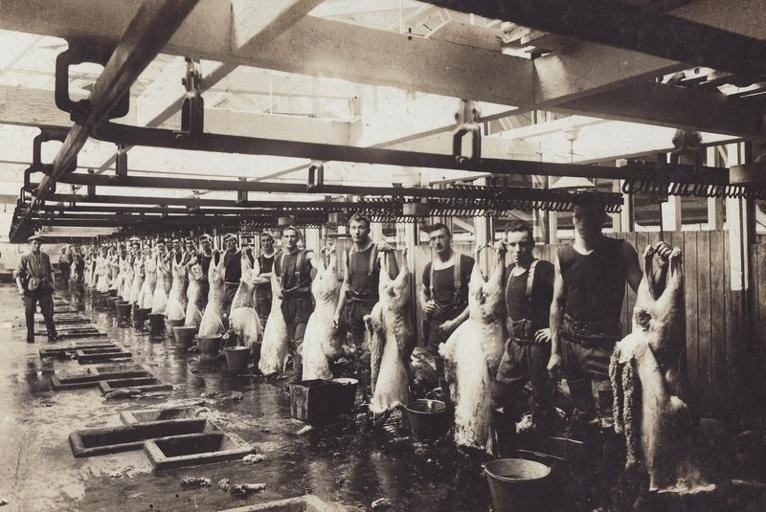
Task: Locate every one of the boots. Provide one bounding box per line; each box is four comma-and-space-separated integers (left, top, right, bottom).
27, 328, 34, 342
48, 328, 65, 341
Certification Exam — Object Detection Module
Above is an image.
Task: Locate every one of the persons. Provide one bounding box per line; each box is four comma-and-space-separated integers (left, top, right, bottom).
486, 219, 561, 457
271, 227, 327, 360
11, 234, 61, 344
330, 213, 400, 402
417, 221, 477, 442
57, 225, 283, 376
545, 187, 674, 476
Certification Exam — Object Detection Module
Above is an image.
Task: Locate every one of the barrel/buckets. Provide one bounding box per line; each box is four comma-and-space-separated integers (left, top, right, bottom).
484, 458, 553, 511
406, 399, 450, 440
94, 287, 250, 376
329, 377, 358, 410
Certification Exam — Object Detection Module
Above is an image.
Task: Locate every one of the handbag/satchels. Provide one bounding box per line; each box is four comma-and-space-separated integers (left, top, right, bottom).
22, 274, 54, 297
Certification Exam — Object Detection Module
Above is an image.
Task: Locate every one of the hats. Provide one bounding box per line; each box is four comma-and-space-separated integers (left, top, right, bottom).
27, 235, 43, 242
571, 191, 605, 208
